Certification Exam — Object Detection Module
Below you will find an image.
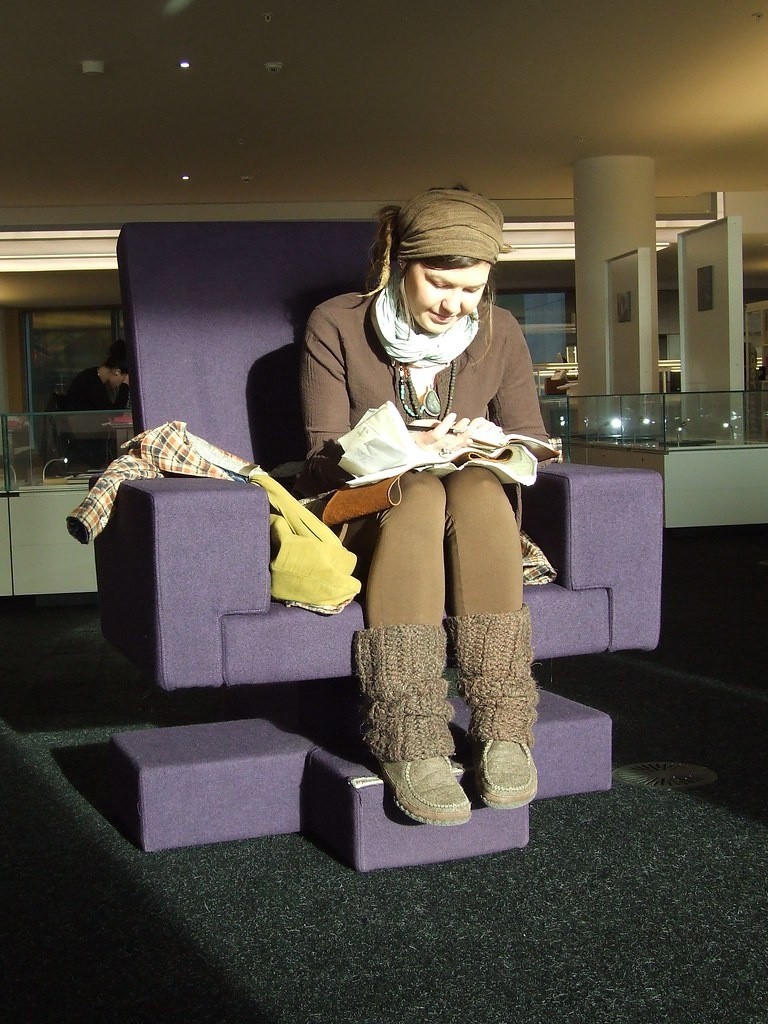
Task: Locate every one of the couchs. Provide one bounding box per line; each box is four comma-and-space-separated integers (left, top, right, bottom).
82, 221, 666, 692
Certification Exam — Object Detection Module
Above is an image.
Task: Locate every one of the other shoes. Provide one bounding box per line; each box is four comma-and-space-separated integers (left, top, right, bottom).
474, 727, 538, 811
377, 754, 472, 827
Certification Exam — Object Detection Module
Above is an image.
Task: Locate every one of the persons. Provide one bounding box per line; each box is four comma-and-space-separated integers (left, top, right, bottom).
53, 338, 130, 471
303, 182, 557, 826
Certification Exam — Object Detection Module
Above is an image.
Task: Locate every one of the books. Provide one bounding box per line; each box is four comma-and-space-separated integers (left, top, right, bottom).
337, 399, 560, 488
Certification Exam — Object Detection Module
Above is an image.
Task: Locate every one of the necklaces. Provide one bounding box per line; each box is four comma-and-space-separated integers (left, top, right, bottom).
399, 358, 457, 421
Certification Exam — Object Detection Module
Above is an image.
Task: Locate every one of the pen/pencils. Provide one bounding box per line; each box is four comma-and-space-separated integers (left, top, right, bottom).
407, 425, 464, 435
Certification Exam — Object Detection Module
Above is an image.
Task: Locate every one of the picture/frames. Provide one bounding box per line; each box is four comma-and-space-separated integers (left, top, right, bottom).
697, 264, 713, 311
